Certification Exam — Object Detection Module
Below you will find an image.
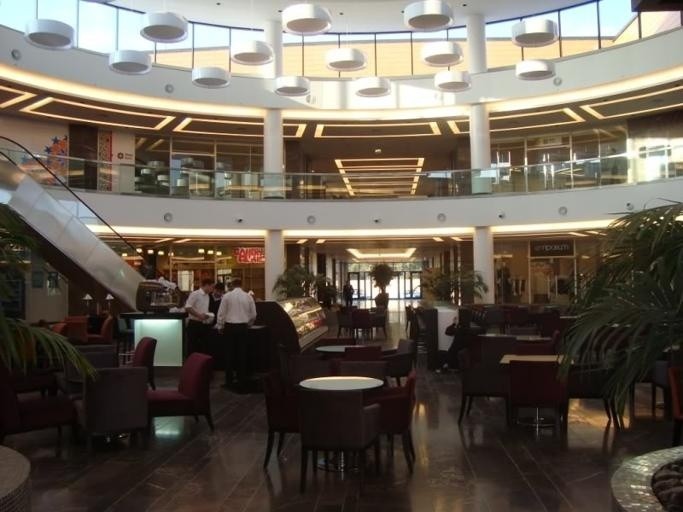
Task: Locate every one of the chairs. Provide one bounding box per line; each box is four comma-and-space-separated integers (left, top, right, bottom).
126, 337, 156, 390
336, 311, 353, 338
342, 306, 358, 311
298, 389, 381, 497
337, 361, 386, 383
50, 323, 67, 337
479, 333, 520, 364
372, 310, 387, 338
365, 369, 417, 474
0, 368, 72, 458
323, 336, 355, 347
262, 368, 309, 470
456, 347, 508, 426
341, 344, 383, 375
75, 367, 150, 456
406, 305, 434, 347
507, 359, 569, 432
552, 330, 559, 350
517, 341, 554, 357
88, 315, 113, 345
461, 305, 489, 335
568, 345, 630, 430
146, 352, 214, 432
352, 309, 373, 339
67, 351, 113, 373
278, 343, 330, 378
380, 339, 415, 385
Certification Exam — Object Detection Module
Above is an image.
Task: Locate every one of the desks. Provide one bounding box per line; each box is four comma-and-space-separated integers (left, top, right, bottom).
316, 345, 365, 352
499, 353, 565, 365
300, 376, 384, 389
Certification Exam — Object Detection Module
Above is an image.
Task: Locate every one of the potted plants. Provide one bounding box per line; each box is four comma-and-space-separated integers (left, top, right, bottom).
558, 201, 683, 512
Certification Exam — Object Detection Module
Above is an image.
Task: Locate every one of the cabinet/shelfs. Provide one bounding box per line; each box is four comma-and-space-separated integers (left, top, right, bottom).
250, 297, 328, 356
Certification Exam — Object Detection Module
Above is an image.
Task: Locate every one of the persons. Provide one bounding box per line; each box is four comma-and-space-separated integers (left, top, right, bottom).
158, 272, 177, 290
186, 279, 216, 359
226, 279, 234, 291
343, 280, 354, 307
611, 148, 621, 184
434, 315, 475, 373
217, 278, 257, 388
247, 289, 262, 301
208, 283, 225, 371
